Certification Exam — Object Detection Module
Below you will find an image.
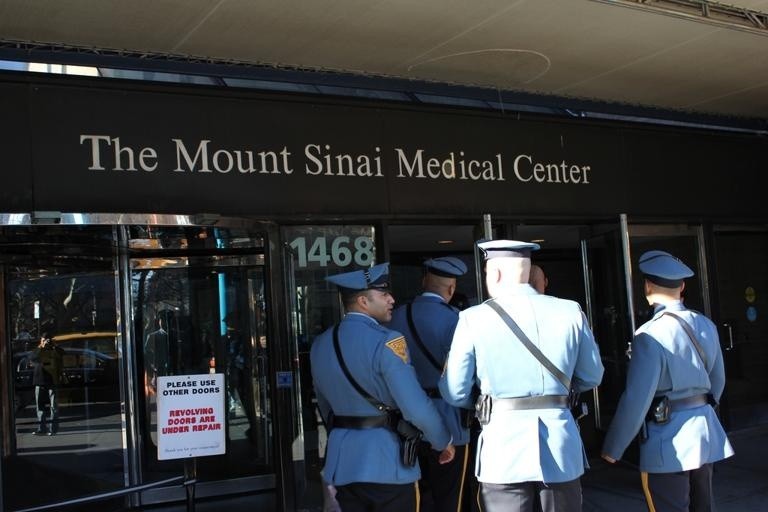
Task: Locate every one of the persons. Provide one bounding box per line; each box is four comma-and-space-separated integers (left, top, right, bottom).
529, 266, 551, 295
599, 250, 736, 512
389, 257, 471, 512
309, 262, 454, 512
144, 310, 258, 426
437, 240, 605, 512
31, 332, 64, 435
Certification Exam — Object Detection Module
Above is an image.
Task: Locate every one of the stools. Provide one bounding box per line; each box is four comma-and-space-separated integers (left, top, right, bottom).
330, 414, 397, 429
489, 394, 571, 413
669, 392, 709, 412
420, 386, 443, 400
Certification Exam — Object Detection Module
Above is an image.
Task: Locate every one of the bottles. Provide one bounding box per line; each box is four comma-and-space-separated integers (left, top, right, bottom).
637, 250, 695, 290
474, 238, 542, 261
323, 262, 391, 291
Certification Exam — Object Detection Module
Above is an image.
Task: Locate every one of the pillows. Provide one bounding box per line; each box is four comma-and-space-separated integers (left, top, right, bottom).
421, 256, 469, 279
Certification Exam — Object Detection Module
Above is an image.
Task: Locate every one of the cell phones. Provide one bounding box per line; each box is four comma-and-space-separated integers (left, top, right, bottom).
46, 338, 53, 345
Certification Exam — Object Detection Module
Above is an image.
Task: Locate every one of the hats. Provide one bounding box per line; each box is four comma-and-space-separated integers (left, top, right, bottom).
8, 330, 120, 414
